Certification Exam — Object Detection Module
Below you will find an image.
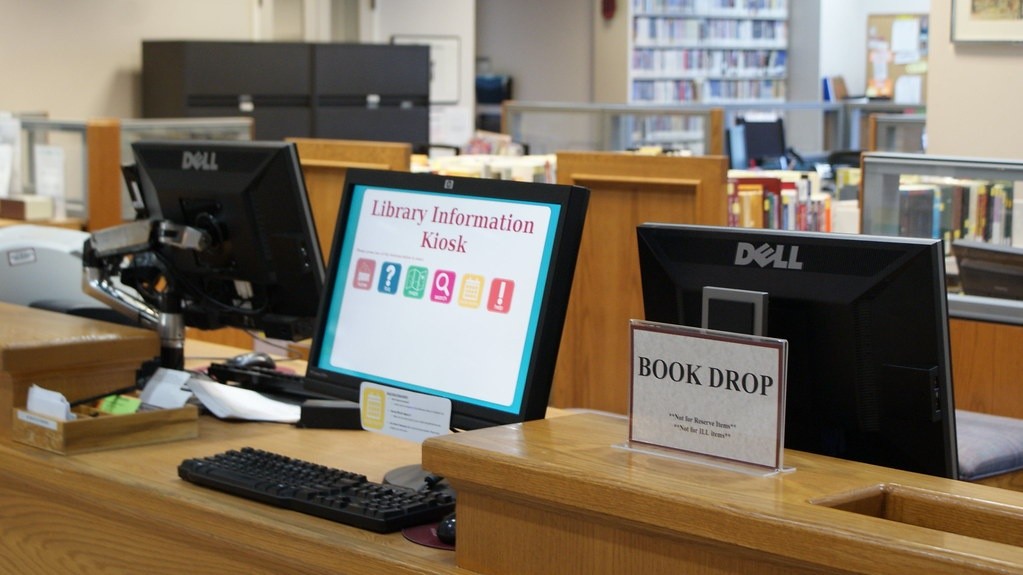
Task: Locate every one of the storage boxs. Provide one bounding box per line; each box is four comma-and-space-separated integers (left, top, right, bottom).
6, 395, 199, 455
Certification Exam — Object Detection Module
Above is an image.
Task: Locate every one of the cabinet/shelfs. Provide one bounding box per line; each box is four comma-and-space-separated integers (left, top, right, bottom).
595, 1, 789, 152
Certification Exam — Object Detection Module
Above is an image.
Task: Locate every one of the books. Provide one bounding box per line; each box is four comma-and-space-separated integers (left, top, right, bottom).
630, 0, 788, 145
727, 167, 1023, 302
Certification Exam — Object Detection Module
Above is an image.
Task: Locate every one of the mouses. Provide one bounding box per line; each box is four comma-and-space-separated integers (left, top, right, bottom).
437, 510, 458, 546
229, 351, 276, 369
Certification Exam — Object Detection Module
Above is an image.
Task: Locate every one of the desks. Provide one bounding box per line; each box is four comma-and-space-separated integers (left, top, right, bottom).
0, 298, 1023, 575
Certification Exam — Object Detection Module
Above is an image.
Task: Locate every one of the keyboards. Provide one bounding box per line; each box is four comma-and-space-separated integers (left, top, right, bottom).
207, 362, 334, 400
177, 447, 456, 533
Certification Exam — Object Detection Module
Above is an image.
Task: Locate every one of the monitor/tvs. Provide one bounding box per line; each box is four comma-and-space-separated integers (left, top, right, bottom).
83, 114, 1023, 506
